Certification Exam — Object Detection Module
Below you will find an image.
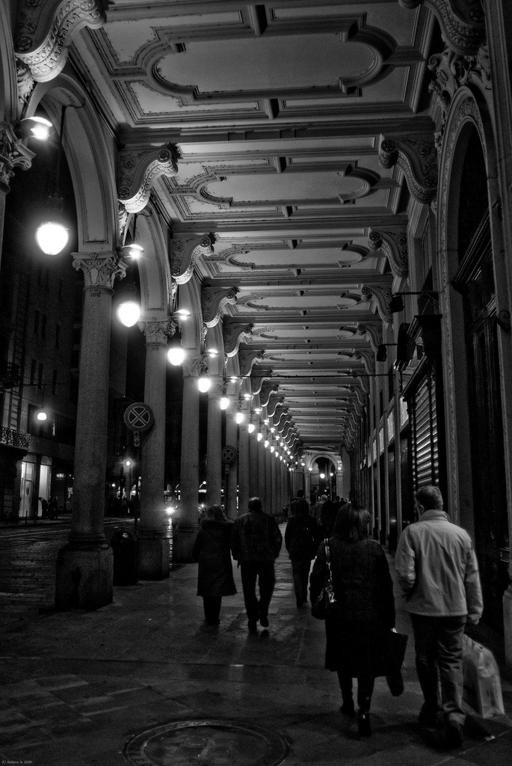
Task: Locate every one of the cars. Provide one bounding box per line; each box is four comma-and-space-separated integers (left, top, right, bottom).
163, 489, 239, 517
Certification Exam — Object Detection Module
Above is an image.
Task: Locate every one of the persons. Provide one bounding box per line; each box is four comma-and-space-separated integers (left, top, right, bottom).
229, 496, 283, 629
393, 485, 486, 728
38, 497, 49, 520
283, 484, 350, 610
190, 503, 237, 626
308, 501, 398, 738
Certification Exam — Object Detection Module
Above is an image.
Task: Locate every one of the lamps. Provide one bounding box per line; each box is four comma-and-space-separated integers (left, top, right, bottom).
196, 362, 211, 394
32, 99, 75, 259
116, 272, 143, 331
168, 327, 189, 371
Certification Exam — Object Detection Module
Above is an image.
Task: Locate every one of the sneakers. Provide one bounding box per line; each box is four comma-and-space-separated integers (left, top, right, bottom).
340, 701, 371, 740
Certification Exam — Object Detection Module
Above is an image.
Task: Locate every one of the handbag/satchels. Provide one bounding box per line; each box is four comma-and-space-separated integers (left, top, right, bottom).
311, 566, 341, 619
460, 634, 506, 721
